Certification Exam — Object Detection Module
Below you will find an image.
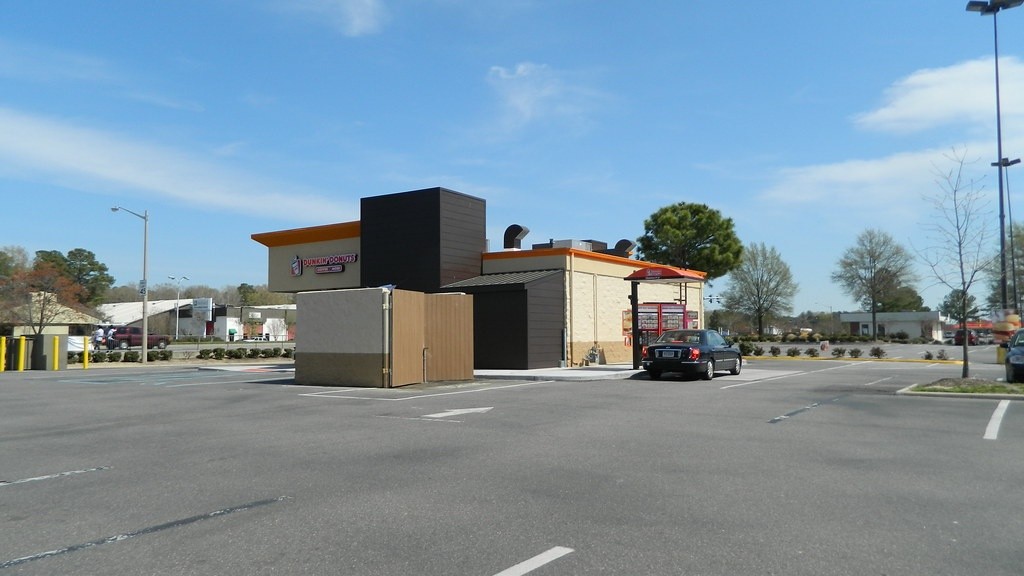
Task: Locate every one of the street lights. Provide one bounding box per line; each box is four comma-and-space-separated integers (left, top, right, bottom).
112, 206, 149, 363
168, 276, 191, 339
991, 158, 1022, 315
965, 0, 1024, 315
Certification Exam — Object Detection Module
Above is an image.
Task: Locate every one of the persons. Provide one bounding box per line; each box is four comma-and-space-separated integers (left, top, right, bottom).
107, 325, 116, 350
94, 326, 104, 350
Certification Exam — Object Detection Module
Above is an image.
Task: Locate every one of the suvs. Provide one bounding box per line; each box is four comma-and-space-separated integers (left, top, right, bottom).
102, 327, 171, 349
243, 337, 268, 342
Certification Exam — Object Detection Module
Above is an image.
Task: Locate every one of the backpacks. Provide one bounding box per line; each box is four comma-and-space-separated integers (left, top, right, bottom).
112, 331, 116, 339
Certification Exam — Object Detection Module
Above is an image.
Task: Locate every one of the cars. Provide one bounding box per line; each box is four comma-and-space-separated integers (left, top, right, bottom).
1000, 328, 1024, 384
642, 329, 742, 380
955, 329, 980, 345
946, 331, 952, 338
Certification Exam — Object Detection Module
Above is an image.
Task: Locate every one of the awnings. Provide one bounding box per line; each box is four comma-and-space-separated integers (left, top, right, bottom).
229, 329, 237, 334
